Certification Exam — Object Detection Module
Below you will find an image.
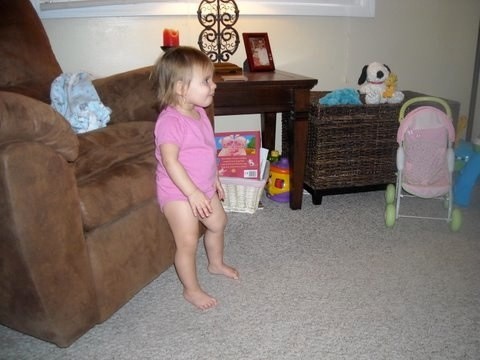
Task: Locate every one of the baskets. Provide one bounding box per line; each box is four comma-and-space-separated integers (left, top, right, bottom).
219, 160, 271, 215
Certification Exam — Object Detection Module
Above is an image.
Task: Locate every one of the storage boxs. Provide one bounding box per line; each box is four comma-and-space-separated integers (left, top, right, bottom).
214, 131, 260, 180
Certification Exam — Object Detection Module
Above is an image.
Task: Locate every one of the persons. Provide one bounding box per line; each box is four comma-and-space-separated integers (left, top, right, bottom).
253, 38, 269, 65
148, 47, 238, 310
217, 133, 248, 156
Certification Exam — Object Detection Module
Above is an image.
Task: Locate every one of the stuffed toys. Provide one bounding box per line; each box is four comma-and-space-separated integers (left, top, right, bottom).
358, 62, 404, 104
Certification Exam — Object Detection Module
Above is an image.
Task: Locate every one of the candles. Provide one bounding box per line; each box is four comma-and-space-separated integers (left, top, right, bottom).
163, 28, 180, 47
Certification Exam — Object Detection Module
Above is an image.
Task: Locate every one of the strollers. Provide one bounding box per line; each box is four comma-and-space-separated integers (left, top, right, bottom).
386, 97, 462, 233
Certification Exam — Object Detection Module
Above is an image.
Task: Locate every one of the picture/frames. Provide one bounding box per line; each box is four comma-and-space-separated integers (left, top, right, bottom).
242, 33, 275, 73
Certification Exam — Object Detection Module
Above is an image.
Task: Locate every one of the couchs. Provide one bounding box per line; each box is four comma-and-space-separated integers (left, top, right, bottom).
0, 0, 210, 348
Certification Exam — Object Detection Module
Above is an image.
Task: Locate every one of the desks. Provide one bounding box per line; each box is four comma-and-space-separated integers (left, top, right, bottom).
212, 70, 318, 211
